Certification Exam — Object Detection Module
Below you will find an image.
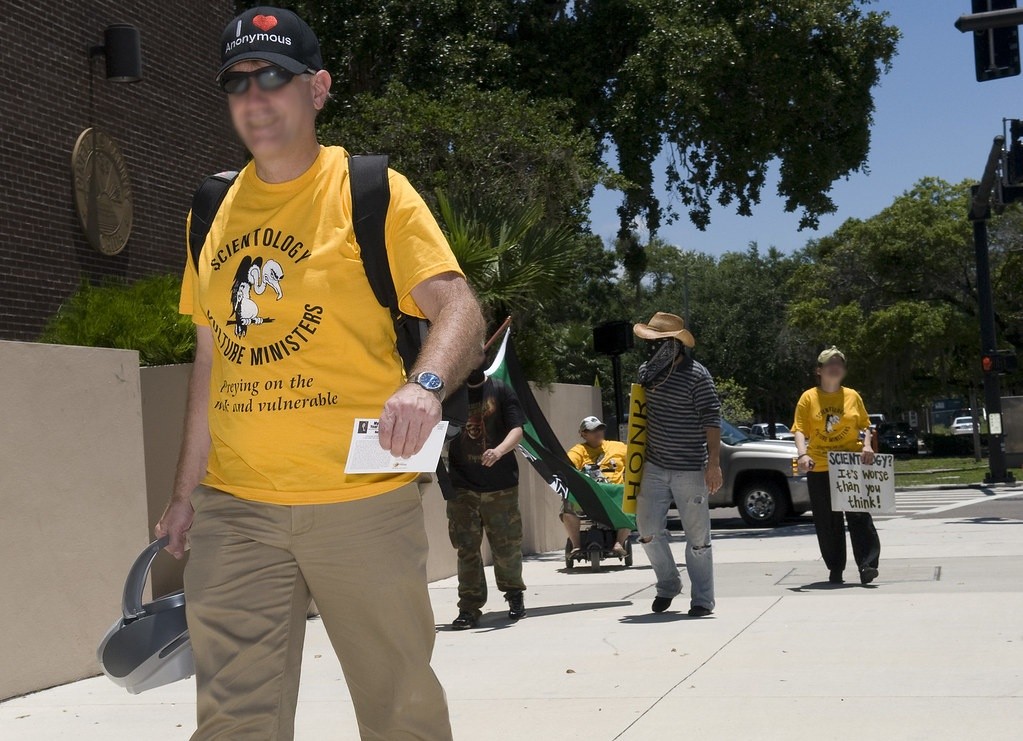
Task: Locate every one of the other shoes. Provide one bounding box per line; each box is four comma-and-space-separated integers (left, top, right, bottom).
652, 596, 673, 612
688, 606, 711, 615
612, 548, 627, 555
570, 545, 582, 556
829, 569, 842, 583
861, 567, 879, 584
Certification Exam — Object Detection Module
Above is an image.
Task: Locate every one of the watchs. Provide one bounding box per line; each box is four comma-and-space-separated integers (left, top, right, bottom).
408, 371, 446, 402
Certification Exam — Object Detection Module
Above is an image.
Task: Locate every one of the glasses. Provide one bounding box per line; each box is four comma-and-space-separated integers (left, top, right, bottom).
219, 65, 312, 96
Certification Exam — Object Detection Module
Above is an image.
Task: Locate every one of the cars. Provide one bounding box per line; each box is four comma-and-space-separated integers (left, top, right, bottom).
950, 416, 981, 435
873, 422, 920, 458
724, 427, 752, 435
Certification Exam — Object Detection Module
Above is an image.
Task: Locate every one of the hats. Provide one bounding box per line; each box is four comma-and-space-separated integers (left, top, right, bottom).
818, 348, 847, 364
216, 6, 322, 82
634, 312, 695, 349
579, 416, 607, 433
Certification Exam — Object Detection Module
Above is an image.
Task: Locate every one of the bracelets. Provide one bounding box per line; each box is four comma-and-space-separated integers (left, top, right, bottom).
796, 453, 808, 459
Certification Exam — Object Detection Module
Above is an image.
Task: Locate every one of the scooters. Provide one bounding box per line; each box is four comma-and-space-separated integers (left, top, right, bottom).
565, 524, 633, 571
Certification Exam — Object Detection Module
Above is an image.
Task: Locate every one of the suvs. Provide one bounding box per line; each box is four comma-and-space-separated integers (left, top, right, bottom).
605, 414, 811, 527
868, 413, 887, 437
749, 424, 795, 441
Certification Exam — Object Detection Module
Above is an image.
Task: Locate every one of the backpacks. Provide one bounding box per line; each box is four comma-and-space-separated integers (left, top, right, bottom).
189, 154, 469, 441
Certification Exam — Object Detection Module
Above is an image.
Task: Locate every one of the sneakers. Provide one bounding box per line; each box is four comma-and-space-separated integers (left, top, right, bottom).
451, 611, 479, 629
508, 593, 527, 620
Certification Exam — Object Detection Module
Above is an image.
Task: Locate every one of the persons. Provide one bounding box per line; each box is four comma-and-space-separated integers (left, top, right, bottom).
437, 334, 528, 629
791, 347, 880, 585
559, 416, 632, 559
155, 8, 486, 741
632, 312, 722, 616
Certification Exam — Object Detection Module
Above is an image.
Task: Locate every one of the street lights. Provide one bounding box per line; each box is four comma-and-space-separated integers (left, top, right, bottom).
592, 320, 635, 440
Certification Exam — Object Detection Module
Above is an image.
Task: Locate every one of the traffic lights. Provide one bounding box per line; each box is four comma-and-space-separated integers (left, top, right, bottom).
982, 351, 1001, 386
1010, 119, 1023, 181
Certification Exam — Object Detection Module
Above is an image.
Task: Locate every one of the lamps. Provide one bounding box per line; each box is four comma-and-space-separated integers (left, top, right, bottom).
88, 23, 142, 83
